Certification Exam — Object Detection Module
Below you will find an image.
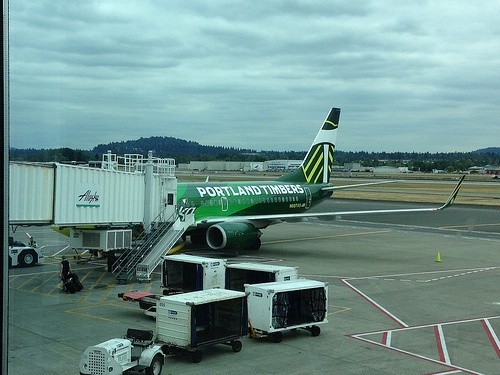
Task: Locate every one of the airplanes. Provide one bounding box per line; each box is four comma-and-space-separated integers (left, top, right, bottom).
49, 108, 466, 256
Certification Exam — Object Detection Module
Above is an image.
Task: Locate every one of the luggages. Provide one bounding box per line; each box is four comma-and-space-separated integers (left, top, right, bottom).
58, 273, 83, 293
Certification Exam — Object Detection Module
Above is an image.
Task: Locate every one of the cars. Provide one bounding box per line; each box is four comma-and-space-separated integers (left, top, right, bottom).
8, 237, 44, 267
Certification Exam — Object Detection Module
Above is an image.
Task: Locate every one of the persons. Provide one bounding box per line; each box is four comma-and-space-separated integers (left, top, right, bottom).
59, 255, 73, 291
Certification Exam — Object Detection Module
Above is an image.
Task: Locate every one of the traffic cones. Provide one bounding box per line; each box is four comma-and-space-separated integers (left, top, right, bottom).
434, 248, 442, 262
